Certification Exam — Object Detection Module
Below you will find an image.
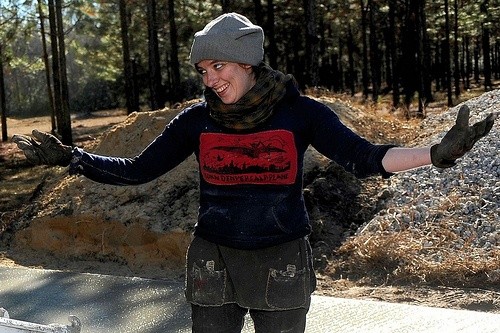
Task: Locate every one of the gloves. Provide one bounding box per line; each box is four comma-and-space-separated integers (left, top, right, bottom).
10, 130, 72, 169
429, 106, 496, 169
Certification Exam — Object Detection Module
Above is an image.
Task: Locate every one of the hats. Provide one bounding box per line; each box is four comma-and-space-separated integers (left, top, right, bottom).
188, 13, 266, 67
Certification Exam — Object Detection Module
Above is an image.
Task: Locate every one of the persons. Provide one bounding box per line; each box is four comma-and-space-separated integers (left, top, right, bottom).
12, 13, 493, 333
